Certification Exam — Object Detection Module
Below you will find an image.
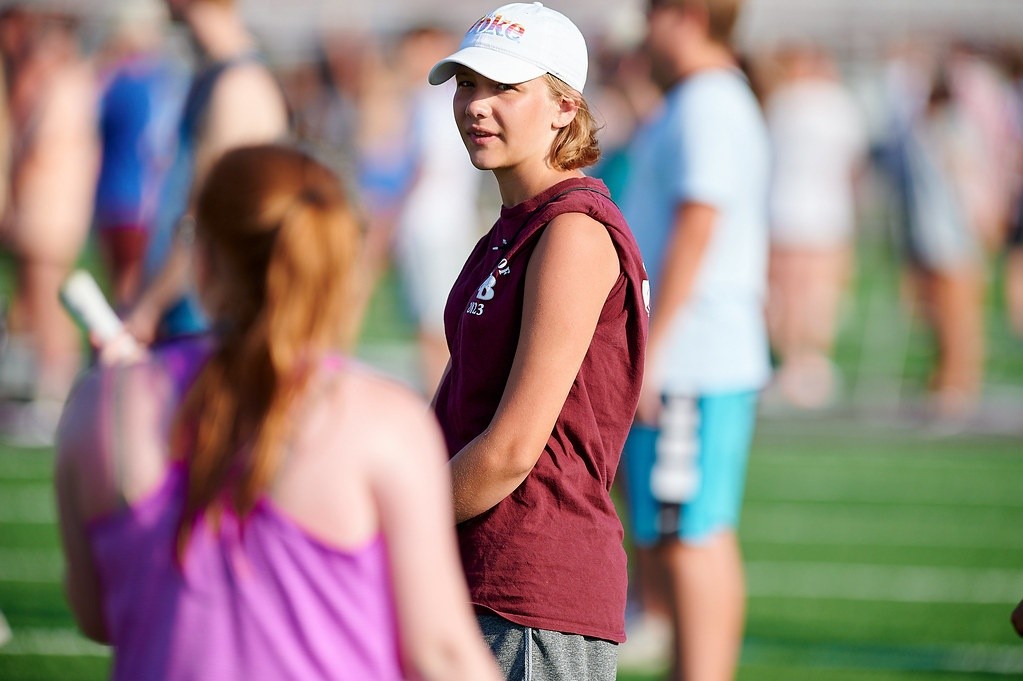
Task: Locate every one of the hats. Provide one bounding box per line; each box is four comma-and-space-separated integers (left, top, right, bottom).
428, 2, 588, 94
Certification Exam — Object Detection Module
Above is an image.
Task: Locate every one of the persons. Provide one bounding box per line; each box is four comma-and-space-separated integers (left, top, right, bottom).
0, 0, 481, 449
761, 45, 867, 407
891, 44, 1023, 418
590, 0, 772, 681
429, 2, 653, 681
53, 144, 505, 681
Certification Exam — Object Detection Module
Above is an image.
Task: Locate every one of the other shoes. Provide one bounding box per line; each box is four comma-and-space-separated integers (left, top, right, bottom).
616, 612, 678, 678
